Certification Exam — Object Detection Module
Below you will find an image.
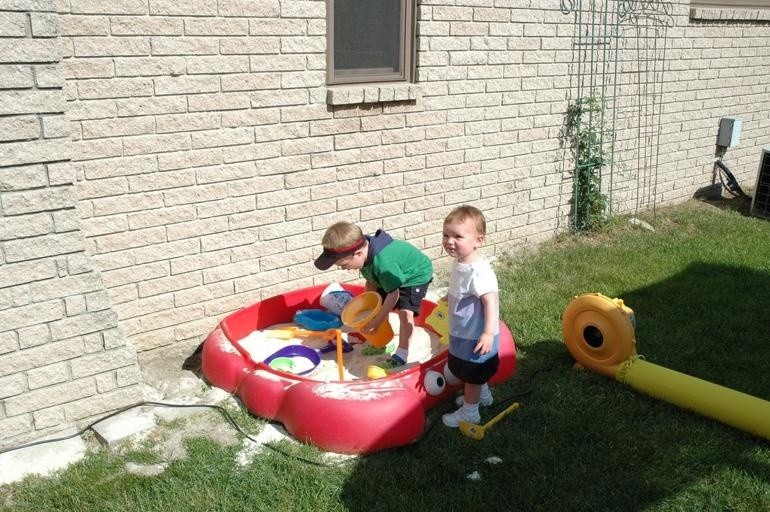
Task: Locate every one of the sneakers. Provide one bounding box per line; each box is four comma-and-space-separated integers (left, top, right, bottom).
382, 354, 406, 369
361, 345, 387, 356
442, 407, 481, 428
455, 390, 494, 408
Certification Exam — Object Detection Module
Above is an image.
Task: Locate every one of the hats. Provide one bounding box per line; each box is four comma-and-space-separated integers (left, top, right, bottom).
314, 236, 365, 271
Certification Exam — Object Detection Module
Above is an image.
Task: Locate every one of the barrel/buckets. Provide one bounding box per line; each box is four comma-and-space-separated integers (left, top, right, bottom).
319, 283, 352, 313
342, 291, 395, 348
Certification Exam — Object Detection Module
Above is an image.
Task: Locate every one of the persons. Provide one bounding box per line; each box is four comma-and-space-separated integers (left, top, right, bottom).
442, 206, 502, 427
314, 222, 435, 369
